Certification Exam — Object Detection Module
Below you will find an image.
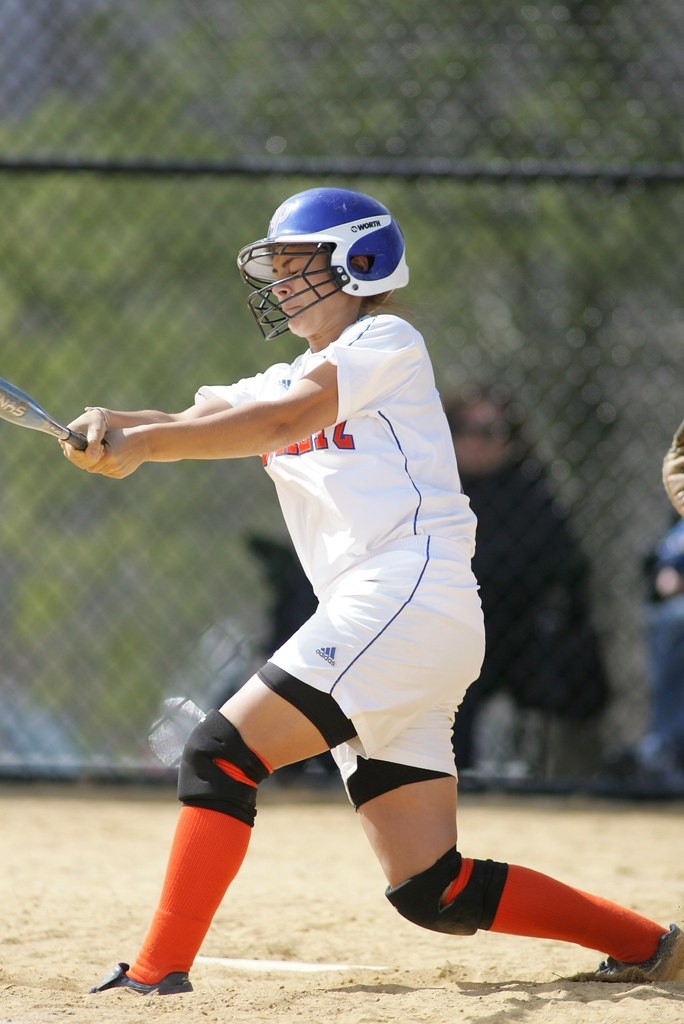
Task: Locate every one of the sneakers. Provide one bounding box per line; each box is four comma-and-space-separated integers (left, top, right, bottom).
88, 962, 193, 995
595, 923, 684, 982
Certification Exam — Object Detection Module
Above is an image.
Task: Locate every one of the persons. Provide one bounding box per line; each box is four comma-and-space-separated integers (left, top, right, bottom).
59, 186, 684, 996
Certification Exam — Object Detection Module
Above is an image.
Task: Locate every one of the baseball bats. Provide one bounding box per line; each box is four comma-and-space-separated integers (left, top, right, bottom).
1, 378, 87, 451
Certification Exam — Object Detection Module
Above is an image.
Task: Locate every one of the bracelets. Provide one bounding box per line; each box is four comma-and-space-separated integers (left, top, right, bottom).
83, 406, 109, 432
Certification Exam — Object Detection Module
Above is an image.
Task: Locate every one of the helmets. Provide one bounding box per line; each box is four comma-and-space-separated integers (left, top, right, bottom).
238, 188, 409, 296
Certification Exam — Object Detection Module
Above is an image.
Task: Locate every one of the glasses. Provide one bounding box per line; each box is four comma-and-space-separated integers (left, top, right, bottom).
453, 418, 512, 439
